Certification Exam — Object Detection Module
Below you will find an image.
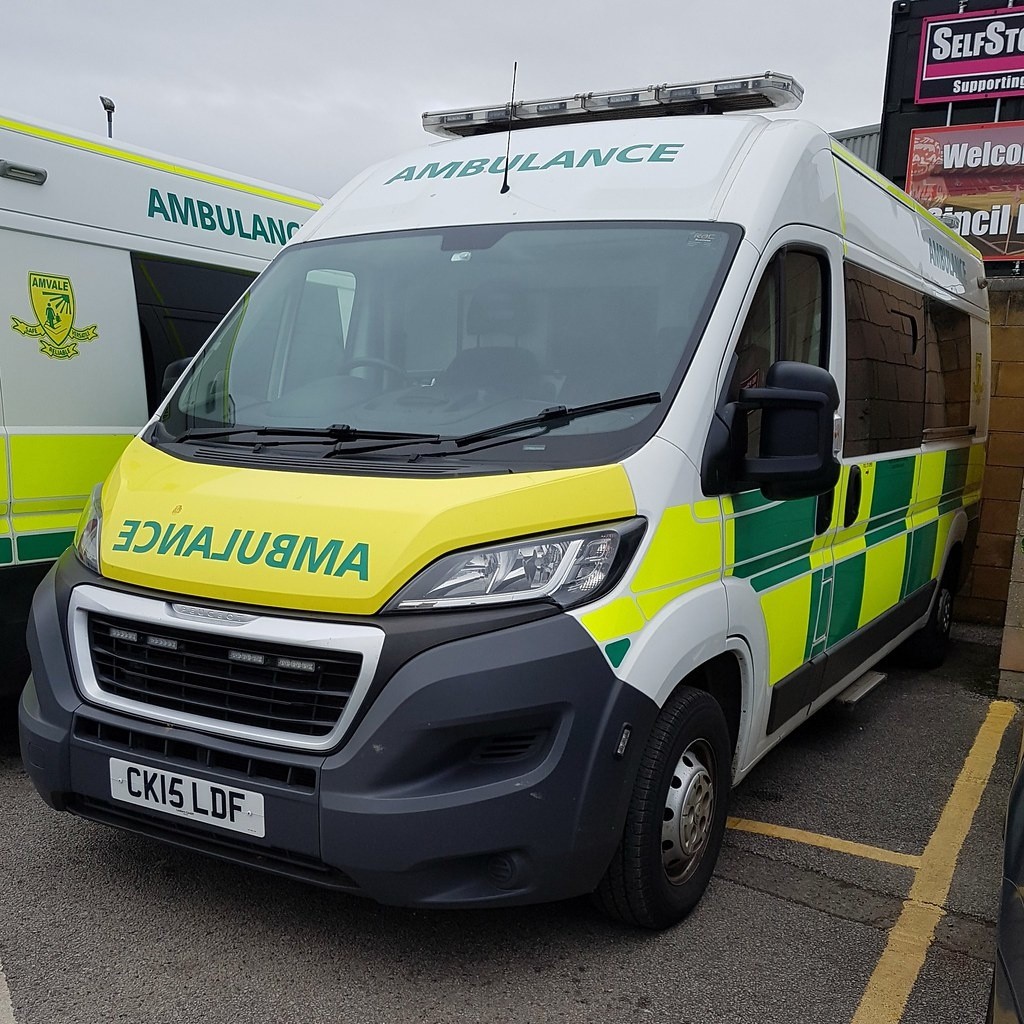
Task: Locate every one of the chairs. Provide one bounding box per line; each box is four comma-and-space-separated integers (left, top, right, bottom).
444, 278, 557, 402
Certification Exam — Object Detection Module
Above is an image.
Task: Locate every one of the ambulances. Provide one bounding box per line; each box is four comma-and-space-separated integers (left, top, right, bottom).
13, 65, 994, 937
1, 112, 357, 719
